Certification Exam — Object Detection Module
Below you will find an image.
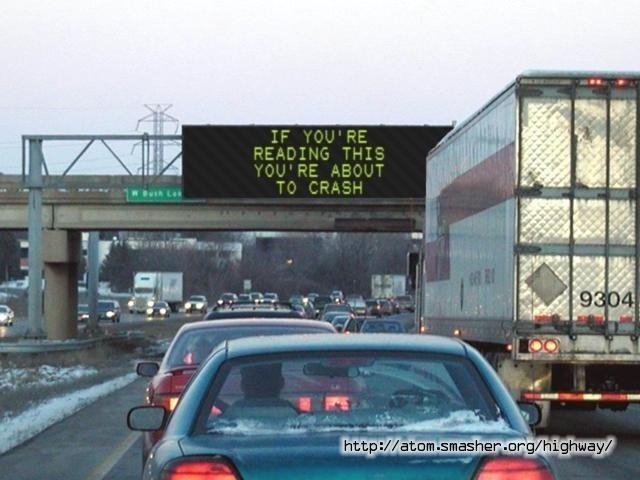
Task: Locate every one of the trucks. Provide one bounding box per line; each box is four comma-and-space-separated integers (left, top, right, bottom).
409, 73, 637, 432
